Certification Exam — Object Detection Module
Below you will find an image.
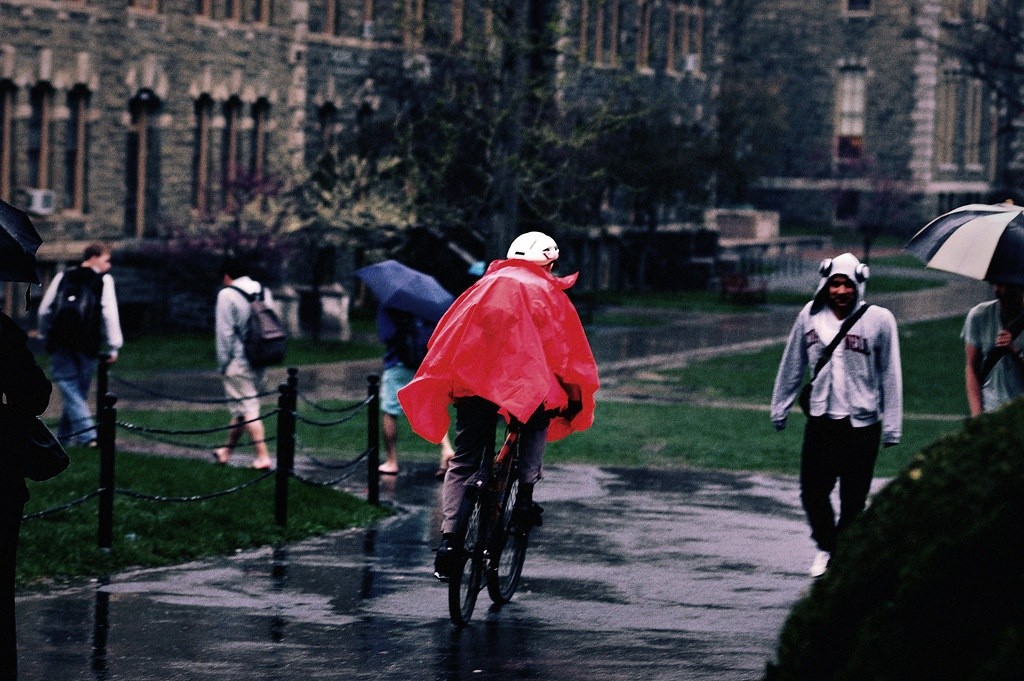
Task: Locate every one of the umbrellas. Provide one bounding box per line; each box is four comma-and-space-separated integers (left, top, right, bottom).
356, 259, 455, 320
901, 200, 1024, 282
0, 199, 44, 286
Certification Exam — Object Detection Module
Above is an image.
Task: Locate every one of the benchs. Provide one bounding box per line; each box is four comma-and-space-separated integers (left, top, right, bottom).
716, 258, 766, 306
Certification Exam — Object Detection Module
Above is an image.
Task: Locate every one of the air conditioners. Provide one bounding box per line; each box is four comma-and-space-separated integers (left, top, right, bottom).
13, 188, 55, 218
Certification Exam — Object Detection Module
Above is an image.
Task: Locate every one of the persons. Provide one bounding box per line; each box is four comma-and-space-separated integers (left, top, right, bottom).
763, 400, 1024, 681
396, 231, 599, 585
767, 251, 904, 576
36, 239, 125, 451
959, 269, 1024, 414
0, 280, 32, 315
0, 312, 70, 681
212, 255, 283, 470
370, 303, 456, 478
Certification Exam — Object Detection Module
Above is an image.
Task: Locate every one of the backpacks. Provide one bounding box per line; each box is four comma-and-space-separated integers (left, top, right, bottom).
48, 264, 109, 349
224, 284, 286, 365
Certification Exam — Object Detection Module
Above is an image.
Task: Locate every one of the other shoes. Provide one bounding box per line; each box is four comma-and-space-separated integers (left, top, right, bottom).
213, 448, 227, 464
811, 551, 832, 576
435, 451, 454, 475
378, 462, 399, 473
253, 458, 271, 469
434, 543, 454, 582
512, 498, 543, 527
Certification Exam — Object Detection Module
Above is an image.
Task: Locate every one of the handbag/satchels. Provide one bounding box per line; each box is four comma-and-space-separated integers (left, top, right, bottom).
9, 399, 70, 481
799, 383, 813, 416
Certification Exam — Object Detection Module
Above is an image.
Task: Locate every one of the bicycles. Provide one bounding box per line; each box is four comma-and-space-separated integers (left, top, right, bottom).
431, 398, 582, 630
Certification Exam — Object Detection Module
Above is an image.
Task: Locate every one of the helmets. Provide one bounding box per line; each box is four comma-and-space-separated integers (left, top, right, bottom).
507, 231, 559, 265
